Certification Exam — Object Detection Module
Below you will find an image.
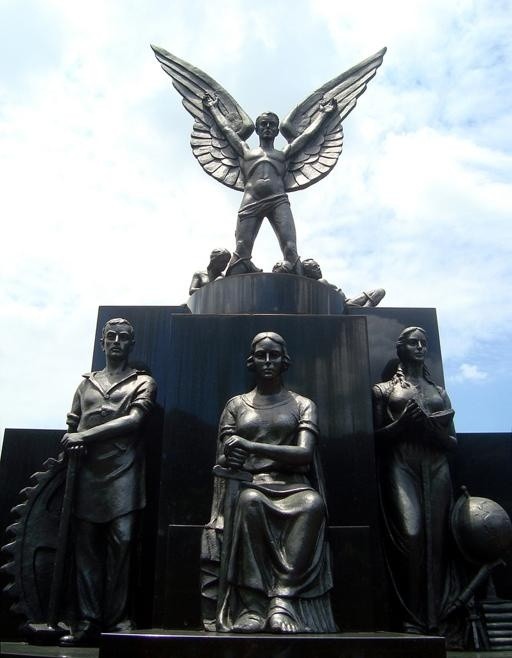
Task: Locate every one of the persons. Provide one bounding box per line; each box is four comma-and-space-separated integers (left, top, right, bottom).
56, 316, 160, 650
199, 89, 338, 274
187, 246, 232, 295
216, 330, 327, 636
370, 324, 460, 635
300, 258, 386, 308
